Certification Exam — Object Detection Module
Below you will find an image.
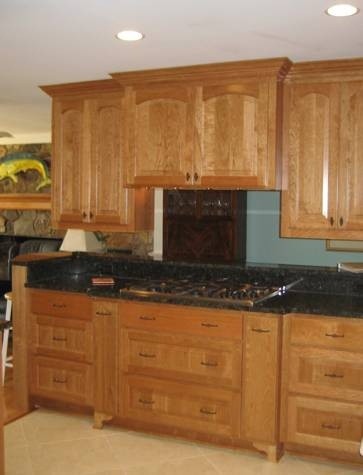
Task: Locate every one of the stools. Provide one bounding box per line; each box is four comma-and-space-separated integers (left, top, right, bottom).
2, 291, 13, 387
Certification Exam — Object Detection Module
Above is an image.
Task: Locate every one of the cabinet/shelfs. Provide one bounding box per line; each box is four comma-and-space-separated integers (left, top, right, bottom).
278, 57, 363, 242
39, 57, 155, 240
110, 58, 294, 191
13, 252, 363, 461
